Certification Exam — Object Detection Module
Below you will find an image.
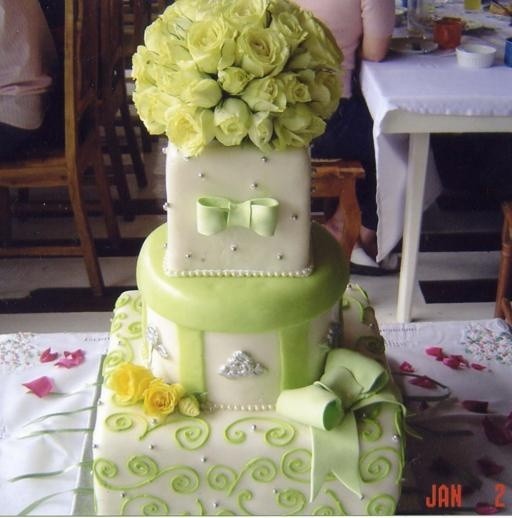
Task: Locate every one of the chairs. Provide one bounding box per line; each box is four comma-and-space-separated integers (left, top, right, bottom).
99, 1, 149, 219
0, 3, 120, 295
122, 3, 158, 150
308, 160, 365, 275
494, 200, 511, 328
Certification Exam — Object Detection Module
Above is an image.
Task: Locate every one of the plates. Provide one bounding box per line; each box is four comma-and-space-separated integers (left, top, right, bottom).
387, 36, 438, 54
455, 44, 496, 68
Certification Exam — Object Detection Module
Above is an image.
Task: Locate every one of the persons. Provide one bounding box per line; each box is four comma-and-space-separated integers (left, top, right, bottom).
287, 0, 403, 277
1, 0, 64, 165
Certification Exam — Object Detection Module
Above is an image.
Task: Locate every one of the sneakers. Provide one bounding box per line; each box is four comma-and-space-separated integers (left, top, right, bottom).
349, 247, 400, 276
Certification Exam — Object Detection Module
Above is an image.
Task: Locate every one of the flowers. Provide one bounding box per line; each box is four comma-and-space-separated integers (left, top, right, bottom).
128, 1, 347, 156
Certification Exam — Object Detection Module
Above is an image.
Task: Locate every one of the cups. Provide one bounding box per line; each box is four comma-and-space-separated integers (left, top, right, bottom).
435, 18, 461, 48
504, 37, 512, 69
465, 0, 481, 12
